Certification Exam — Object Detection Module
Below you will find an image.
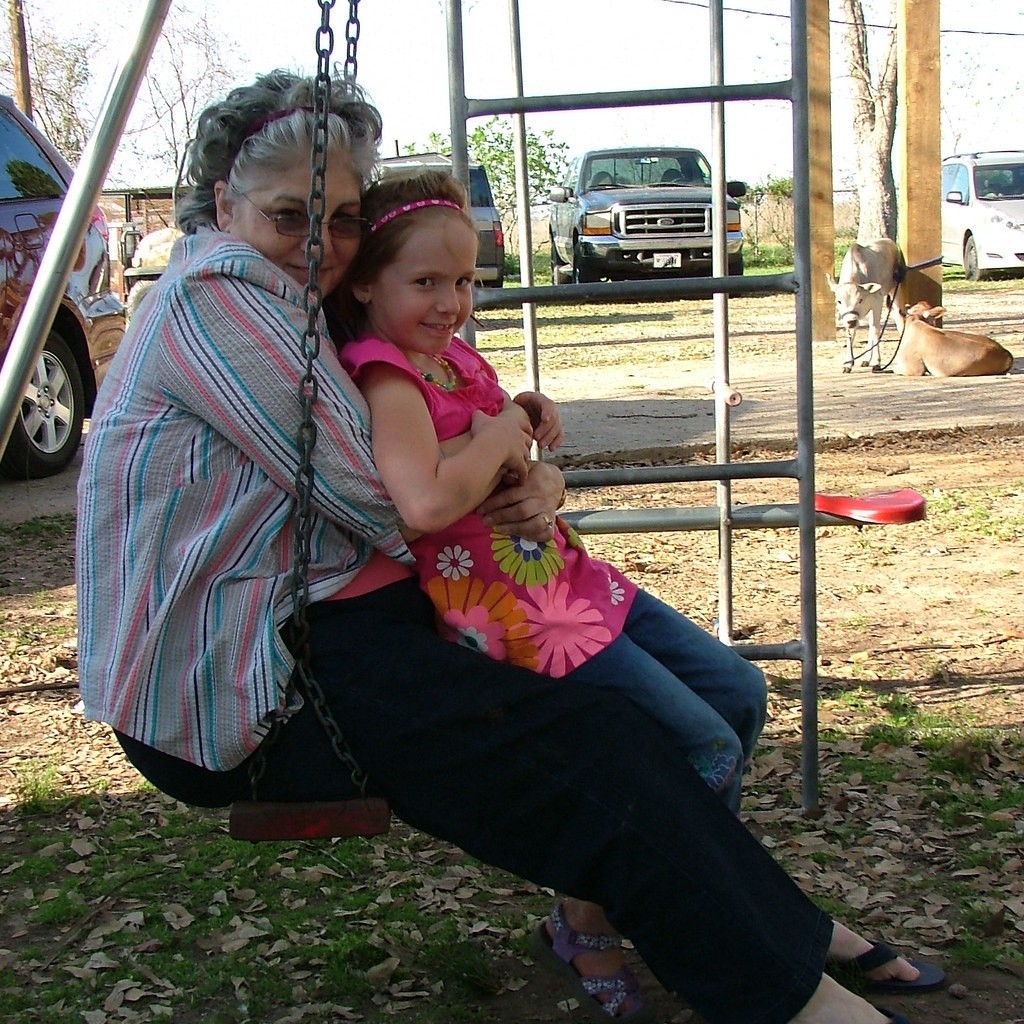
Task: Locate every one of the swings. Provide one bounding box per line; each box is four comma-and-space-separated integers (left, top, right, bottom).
224, 0, 391, 841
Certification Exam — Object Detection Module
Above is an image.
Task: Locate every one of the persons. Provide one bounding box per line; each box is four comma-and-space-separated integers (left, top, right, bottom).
78, 70, 957, 1023
322, 173, 768, 1023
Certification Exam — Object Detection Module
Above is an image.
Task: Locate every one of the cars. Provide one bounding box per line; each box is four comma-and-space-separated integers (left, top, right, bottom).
1, 91, 135, 484
364, 157, 511, 290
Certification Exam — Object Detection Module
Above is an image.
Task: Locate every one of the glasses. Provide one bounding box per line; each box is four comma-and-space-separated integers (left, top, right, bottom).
229, 176, 370, 241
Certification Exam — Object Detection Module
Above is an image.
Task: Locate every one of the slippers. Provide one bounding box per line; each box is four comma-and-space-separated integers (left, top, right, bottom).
836, 919, 946, 997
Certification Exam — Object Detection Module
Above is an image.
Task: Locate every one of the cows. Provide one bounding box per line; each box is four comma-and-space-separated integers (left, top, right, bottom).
825, 236, 908, 375
895, 300, 1015, 377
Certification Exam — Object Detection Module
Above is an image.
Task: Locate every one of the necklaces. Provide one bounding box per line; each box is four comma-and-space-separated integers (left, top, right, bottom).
417, 352, 455, 392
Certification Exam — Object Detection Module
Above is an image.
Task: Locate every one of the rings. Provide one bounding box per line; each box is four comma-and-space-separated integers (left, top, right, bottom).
541, 515, 553, 530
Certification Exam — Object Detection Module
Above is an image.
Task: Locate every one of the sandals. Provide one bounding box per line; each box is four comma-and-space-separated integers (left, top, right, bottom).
528, 904, 644, 1024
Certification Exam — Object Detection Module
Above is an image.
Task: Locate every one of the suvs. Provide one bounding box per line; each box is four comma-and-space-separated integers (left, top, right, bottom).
541, 140, 749, 290
940, 143, 1024, 284
